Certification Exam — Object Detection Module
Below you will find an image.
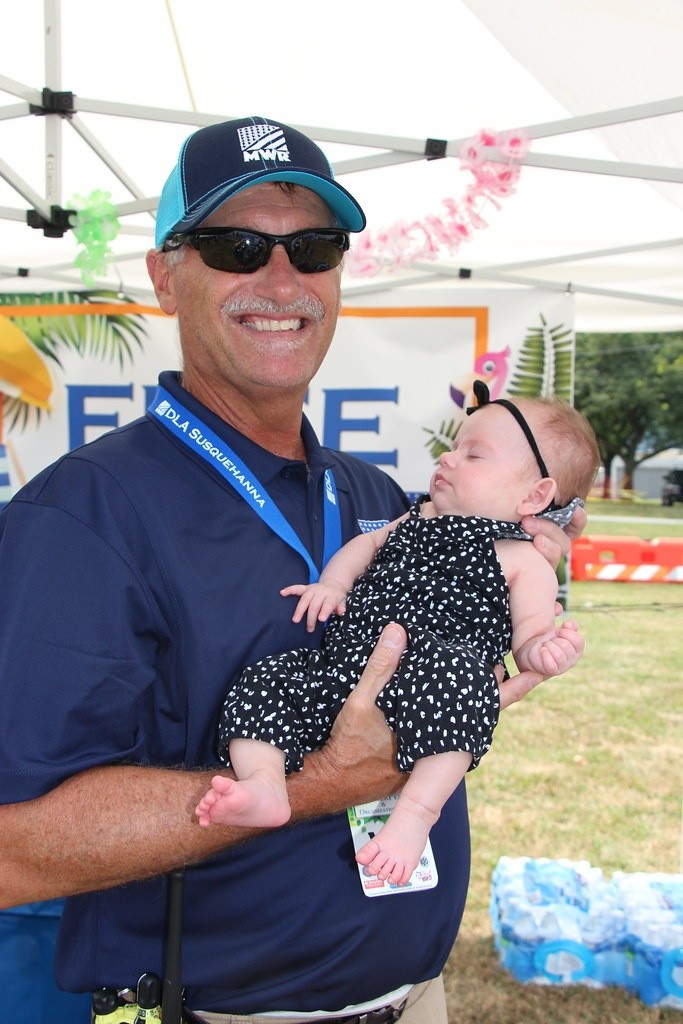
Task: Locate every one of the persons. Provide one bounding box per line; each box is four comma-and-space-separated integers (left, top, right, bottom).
196, 380, 600, 887
0, 117, 589, 1023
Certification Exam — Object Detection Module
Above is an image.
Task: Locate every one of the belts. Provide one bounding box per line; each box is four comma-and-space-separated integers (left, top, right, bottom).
312, 1000, 409, 1024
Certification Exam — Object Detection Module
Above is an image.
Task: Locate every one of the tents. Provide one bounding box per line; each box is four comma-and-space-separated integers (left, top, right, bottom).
0, 0, 682, 335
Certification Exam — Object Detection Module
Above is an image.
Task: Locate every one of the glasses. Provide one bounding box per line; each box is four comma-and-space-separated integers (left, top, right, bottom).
190, 227, 352, 273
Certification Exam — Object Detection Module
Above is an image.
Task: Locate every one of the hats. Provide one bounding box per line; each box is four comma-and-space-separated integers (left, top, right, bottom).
154, 117, 363, 261
